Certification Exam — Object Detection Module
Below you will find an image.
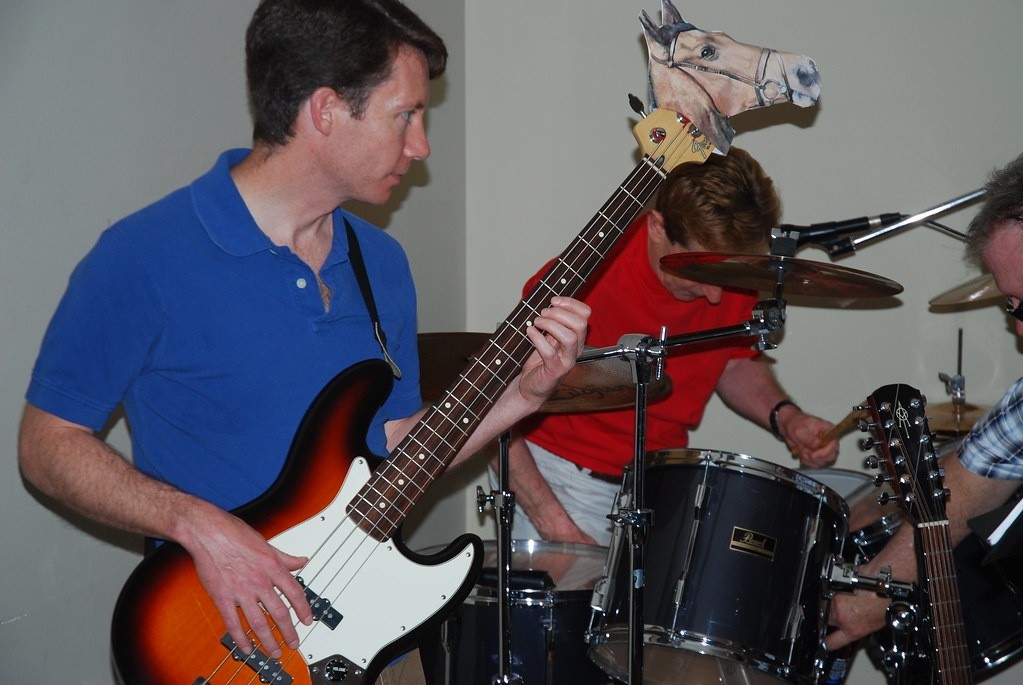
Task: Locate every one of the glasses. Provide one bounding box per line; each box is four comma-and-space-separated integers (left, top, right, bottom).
1004, 295, 1023, 323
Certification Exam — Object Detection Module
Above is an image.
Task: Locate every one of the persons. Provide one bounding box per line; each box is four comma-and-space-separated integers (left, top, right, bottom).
17, 0, 592, 659
483, 146, 840, 546
824, 151, 1023, 651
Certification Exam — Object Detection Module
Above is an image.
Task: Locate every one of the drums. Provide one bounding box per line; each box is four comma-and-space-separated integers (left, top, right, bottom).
582, 447, 851, 685
839, 437, 1023, 685
411, 537, 611, 685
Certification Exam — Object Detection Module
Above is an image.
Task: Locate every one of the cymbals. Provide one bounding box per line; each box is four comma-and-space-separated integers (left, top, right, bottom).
659, 251, 905, 296
929, 273, 1004, 306
418, 331, 673, 414
927, 401, 991, 434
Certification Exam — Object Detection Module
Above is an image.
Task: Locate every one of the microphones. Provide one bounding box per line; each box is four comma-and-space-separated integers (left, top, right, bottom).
781, 212, 900, 248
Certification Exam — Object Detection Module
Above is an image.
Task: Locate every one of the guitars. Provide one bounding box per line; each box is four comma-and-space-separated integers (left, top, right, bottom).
852, 382, 974, 685
109, 93, 713, 685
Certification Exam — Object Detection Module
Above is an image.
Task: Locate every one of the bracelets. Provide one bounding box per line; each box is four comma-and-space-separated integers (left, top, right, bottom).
768, 399, 801, 441
